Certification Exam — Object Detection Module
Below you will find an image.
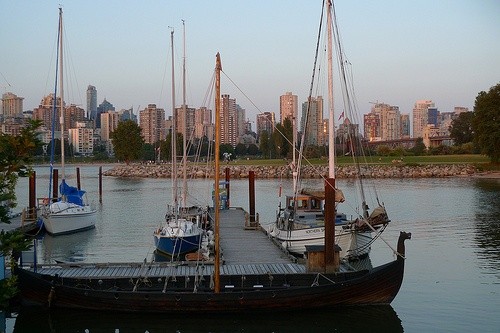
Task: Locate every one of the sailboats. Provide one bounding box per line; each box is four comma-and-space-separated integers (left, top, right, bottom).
15, 52, 412, 313
37, 7, 95, 235
153, 30, 203, 255
271, 2, 390, 262
166, 20, 208, 224
